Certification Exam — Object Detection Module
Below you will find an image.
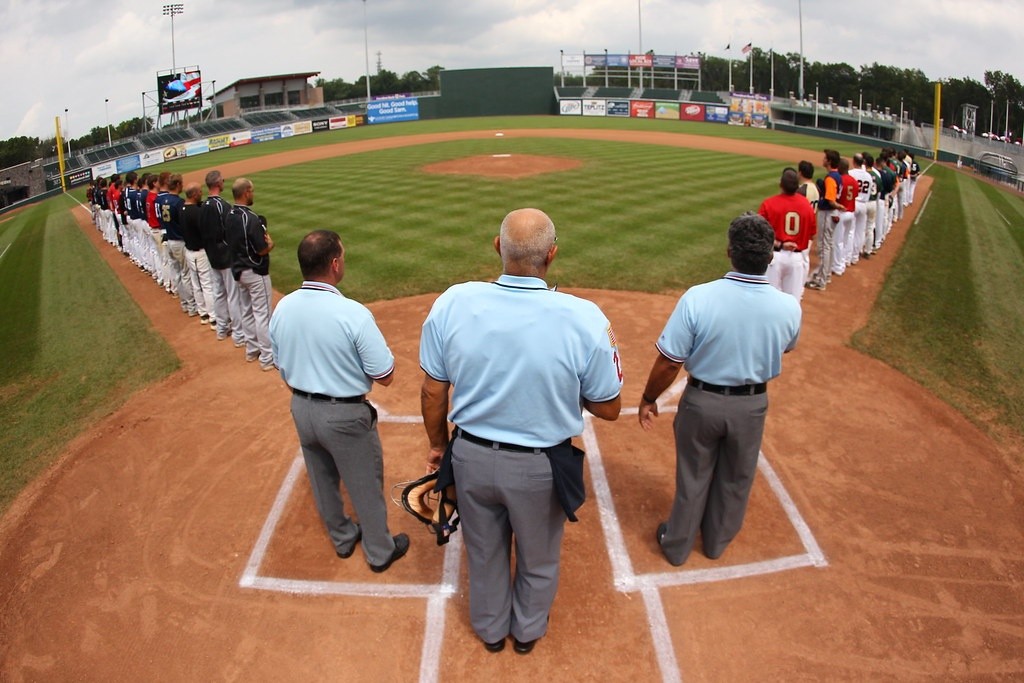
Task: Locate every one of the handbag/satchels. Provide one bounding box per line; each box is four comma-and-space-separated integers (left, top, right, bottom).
546, 445, 586, 522
433, 430, 455, 493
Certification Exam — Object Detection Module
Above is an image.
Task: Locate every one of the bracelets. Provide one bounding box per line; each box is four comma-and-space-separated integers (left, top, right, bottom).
779, 242, 784, 248
643, 394, 655, 403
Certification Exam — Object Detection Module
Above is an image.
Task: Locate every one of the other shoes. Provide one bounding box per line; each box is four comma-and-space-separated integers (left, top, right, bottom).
103, 236, 274, 371
485, 636, 505, 652
657, 522, 667, 543
804, 218, 897, 290
514, 612, 550, 653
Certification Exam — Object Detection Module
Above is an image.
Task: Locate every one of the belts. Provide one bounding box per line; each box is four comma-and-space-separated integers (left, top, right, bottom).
456, 427, 572, 453
688, 375, 766, 396
292, 388, 365, 403
774, 247, 801, 252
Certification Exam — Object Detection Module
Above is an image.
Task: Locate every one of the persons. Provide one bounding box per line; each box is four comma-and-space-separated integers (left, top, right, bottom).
638, 212, 802, 566
225, 178, 274, 371
87, 170, 217, 331
862, 147, 920, 260
805, 148, 846, 291
419, 207, 624, 655
199, 170, 245, 347
757, 167, 817, 303
831, 158, 859, 276
798, 161, 820, 300
270, 229, 410, 574
846, 153, 872, 267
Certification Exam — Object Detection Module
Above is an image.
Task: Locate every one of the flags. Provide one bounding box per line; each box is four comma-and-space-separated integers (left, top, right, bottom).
741, 43, 751, 54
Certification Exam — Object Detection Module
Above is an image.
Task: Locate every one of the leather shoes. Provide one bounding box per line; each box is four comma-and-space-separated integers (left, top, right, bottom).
370, 532, 410, 573
335, 522, 362, 558
